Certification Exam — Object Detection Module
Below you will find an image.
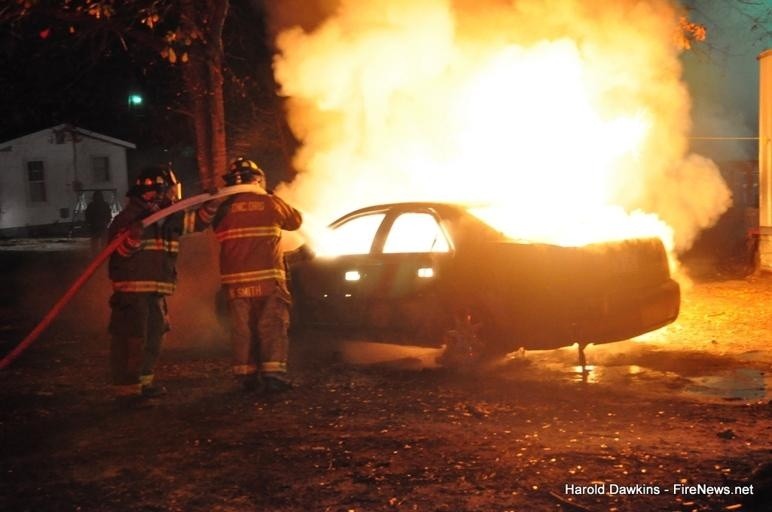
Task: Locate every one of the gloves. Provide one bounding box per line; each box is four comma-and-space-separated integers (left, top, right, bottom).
202, 188, 222, 214
129, 219, 143, 241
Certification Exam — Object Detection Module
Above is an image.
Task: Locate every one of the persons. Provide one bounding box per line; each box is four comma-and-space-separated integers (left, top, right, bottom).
107, 173, 220, 409
85, 192, 111, 254
197, 159, 302, 390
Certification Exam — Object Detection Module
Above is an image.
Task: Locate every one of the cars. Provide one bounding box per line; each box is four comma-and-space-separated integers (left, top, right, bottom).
216, 200, 680, 349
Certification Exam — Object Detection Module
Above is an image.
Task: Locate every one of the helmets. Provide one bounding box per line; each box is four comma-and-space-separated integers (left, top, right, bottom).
126, 165, 182, 202
221, 158, 264, 184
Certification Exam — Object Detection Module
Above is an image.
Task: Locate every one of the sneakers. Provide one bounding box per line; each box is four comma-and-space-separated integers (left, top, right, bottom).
141, 385, 166, 398
120, 393, 154, 409
245, 377, 291, 394
